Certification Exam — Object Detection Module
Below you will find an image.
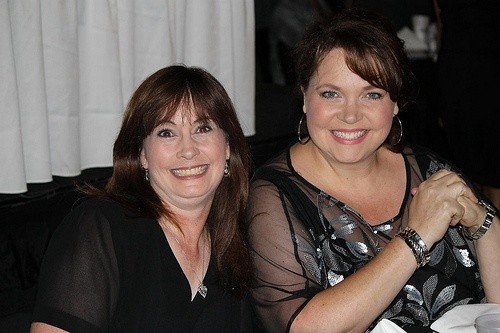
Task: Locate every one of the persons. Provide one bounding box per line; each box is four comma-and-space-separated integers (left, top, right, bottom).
27, 63, 262, 333
238, 19, 500, 333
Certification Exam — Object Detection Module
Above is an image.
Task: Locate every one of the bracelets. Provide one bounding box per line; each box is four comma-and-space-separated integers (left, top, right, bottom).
394, 223, 432, 270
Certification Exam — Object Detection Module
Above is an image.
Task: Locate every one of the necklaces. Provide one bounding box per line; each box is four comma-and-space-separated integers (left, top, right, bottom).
164, 223, 209, 300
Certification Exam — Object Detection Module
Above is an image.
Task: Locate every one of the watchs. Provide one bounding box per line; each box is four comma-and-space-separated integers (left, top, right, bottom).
461, 197, 499, 243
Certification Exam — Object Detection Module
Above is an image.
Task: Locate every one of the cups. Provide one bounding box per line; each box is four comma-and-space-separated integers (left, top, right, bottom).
475, 313, 500, 333
